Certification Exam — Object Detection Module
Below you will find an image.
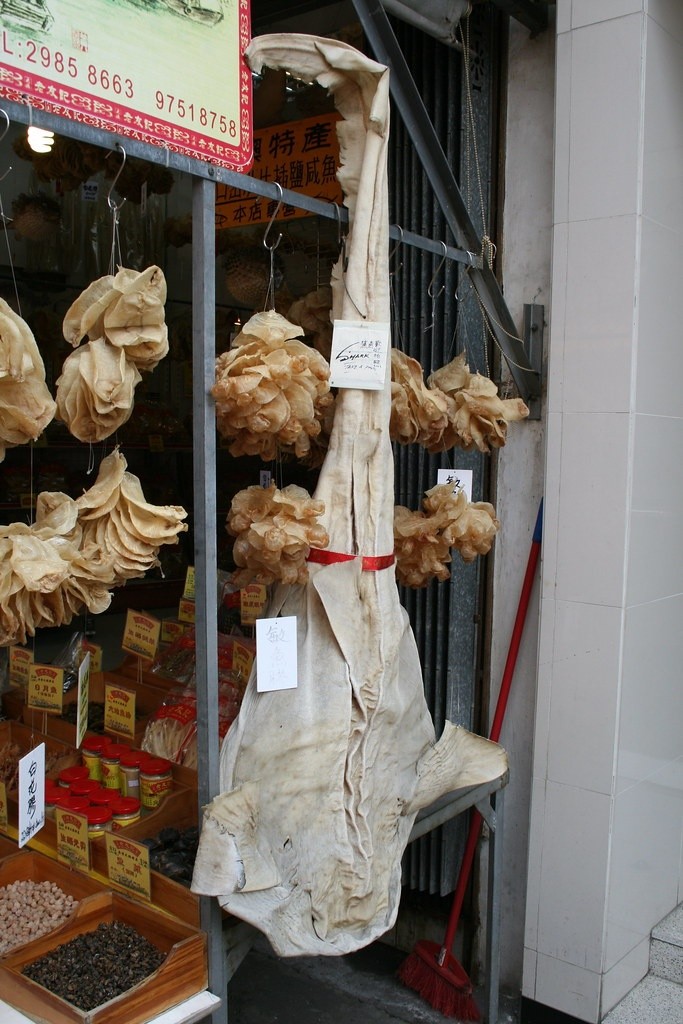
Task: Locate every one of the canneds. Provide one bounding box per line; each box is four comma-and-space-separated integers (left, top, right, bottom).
44, 734, 173, 839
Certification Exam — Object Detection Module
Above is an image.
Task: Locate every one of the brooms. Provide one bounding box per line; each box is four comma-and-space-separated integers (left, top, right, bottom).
395, 492, 549, 1024
